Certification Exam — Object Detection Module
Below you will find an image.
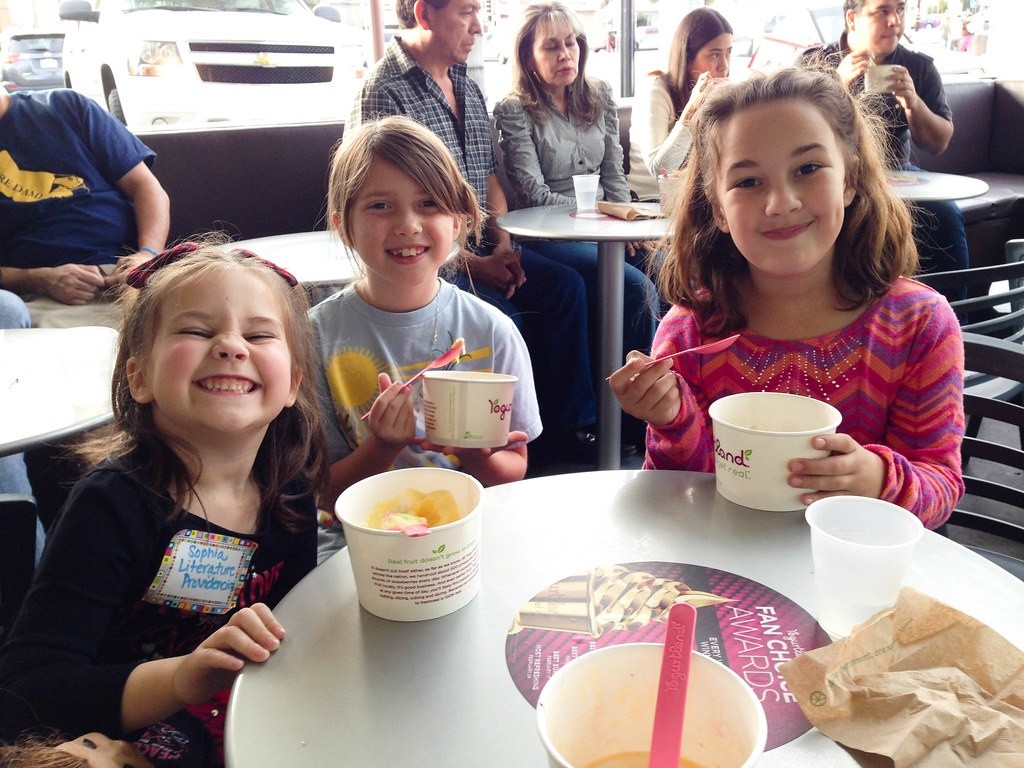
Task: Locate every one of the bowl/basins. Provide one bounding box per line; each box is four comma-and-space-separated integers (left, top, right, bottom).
709, 392, 842, 511
335, 467, 485, 622
536, 643, 768, 768
707, 78, 731, 94
865, 65, 903, 93
422, 370, 519, 448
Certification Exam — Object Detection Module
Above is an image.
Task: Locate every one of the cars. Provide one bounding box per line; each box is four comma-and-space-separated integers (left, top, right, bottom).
479, 0, 1001, 91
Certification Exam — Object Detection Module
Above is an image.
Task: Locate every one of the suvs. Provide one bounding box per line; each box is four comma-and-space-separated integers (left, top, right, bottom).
0, 0, 371, 129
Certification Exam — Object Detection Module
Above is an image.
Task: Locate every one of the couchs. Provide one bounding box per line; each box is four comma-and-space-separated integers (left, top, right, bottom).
111, 80, 1024, 459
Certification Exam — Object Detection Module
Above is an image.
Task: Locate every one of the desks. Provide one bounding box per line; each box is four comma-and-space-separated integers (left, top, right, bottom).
883, 170, 990, 202
222, 470, 1024, 768
495, 201, 677, 470
0, 326, 132, 643
199, 230, 462, 308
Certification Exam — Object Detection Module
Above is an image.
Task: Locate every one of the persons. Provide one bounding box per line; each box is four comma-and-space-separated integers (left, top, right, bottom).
793, 0, 969, 327
0, 246, 329, 745
302, 116, 544, 567
626, 7, 733, 331
493, 2, 674, 459
342, 0, 598, 465
609, 66, 966, 530
0, 78, 170, 306
0, 688, 231, 768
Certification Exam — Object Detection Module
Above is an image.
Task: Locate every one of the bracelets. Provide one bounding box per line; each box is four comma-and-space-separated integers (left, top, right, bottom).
138, 247, 157, 255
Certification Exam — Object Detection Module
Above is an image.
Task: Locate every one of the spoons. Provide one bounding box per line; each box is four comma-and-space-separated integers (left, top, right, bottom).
606, 334, 741, 381
361, 338, 464, 421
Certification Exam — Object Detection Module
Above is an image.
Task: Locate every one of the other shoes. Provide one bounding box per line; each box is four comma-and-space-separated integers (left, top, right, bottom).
543, 431, 638, 464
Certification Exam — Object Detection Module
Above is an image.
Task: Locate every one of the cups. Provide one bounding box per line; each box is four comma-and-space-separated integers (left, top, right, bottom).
658, 174, 678, 213
572, 175, 600, 213
805, 495, 924, 640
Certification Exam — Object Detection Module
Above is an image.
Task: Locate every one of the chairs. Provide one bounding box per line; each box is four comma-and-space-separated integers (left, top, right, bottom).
932, 330, 1024, 582
909, 237, 1024, 470
